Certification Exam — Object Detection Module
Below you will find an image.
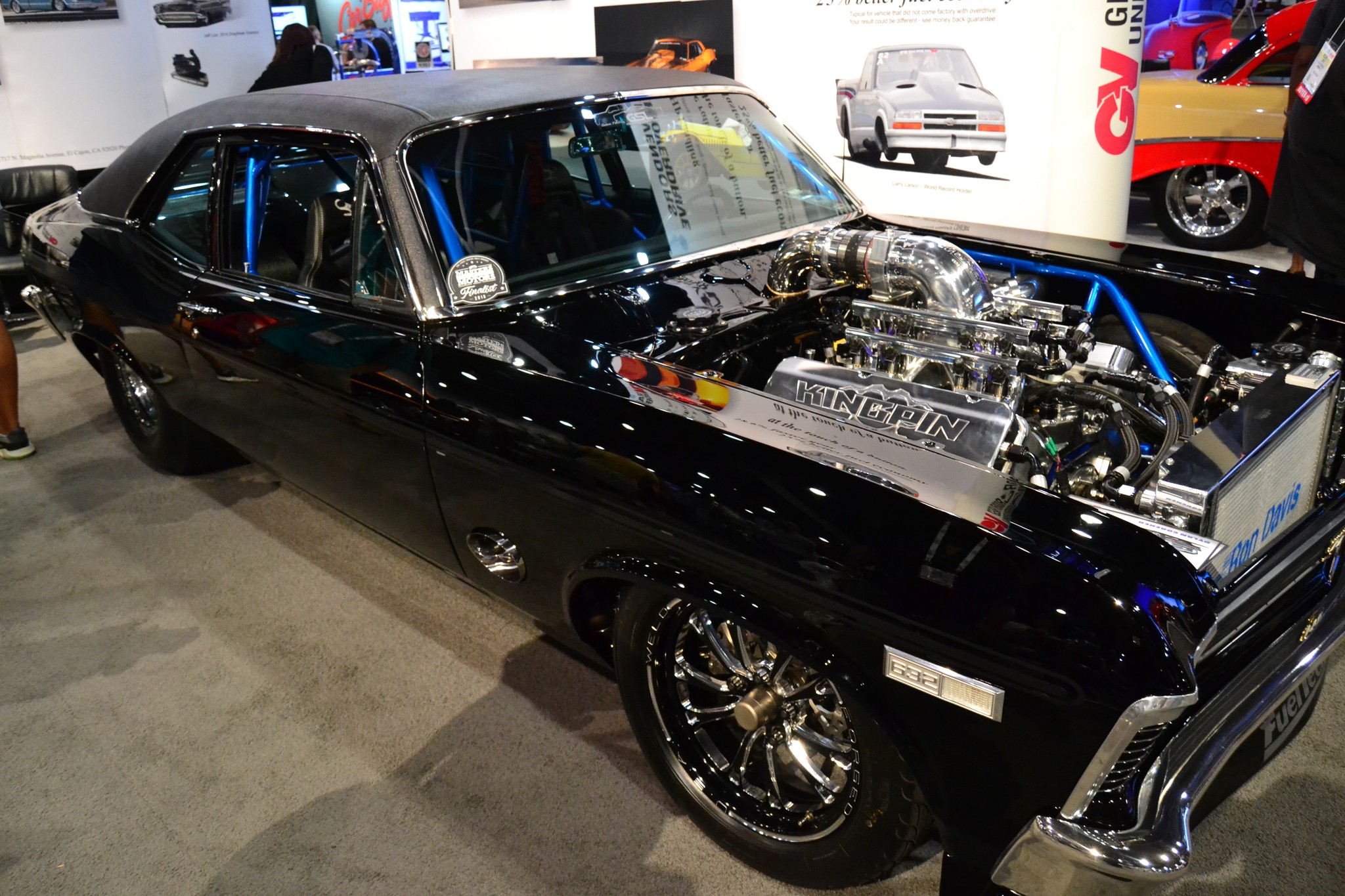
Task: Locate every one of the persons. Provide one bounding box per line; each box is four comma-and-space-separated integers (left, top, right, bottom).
247, 22, 334, 93
342, 18, 394, 68
1261, 0, 1345, 285
306, 24, 341, 81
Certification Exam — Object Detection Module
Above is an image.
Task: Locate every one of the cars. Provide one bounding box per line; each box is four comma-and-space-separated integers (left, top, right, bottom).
152, 1, 231, 28
0, 0, 114, 13
833, 45, 1005, 171
18, 64, 1345, 895
626, 38, 718, 73
1131, 0, 1323, 250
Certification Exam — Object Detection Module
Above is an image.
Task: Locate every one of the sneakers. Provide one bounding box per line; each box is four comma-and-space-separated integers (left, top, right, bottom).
0, 428, 37, 459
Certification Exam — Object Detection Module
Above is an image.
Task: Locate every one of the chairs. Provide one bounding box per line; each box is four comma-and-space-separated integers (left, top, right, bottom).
516, 160, 635, 273
297, 193, 389, 299
0, 165, 76, 317
